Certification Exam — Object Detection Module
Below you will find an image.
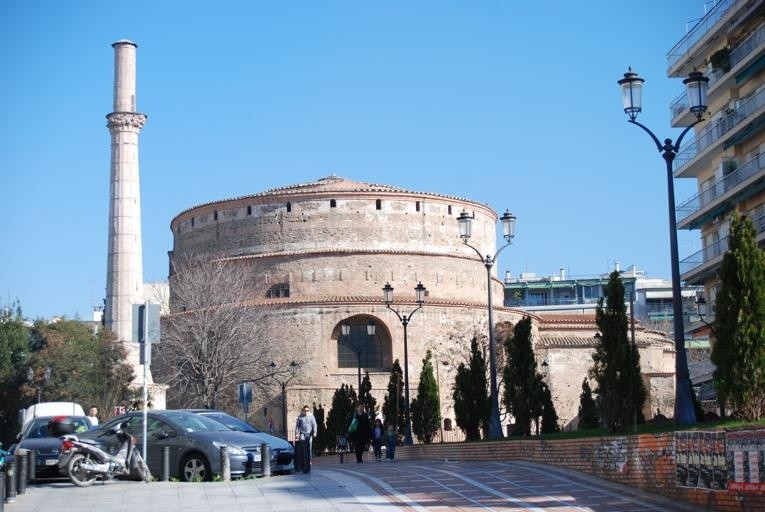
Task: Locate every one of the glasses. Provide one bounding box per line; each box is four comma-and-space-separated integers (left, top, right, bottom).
304, 409, 310, 413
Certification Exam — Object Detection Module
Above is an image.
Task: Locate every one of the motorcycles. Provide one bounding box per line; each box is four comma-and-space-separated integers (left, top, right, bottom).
61, 416, 153, 486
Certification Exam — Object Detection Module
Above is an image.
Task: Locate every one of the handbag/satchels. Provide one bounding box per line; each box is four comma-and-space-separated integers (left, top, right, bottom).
347, 408, 359, 433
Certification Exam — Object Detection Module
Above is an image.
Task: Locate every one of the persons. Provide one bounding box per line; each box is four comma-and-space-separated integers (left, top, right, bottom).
295, 405, 318, 464
345, 402, 372, 463
85, 406, 98, 427
371, 419, 385, 458
384, 425, 398, 462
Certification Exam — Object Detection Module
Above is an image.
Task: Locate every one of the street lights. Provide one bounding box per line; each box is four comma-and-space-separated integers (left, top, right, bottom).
452, 207, 518, 445
615, 68, 718, 427
380, 280, 427, 445
25, 365, 52, 403
266, 359, 299, 441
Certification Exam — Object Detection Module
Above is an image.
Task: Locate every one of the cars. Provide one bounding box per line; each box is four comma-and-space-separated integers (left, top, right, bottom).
0, 392, 295, 482
